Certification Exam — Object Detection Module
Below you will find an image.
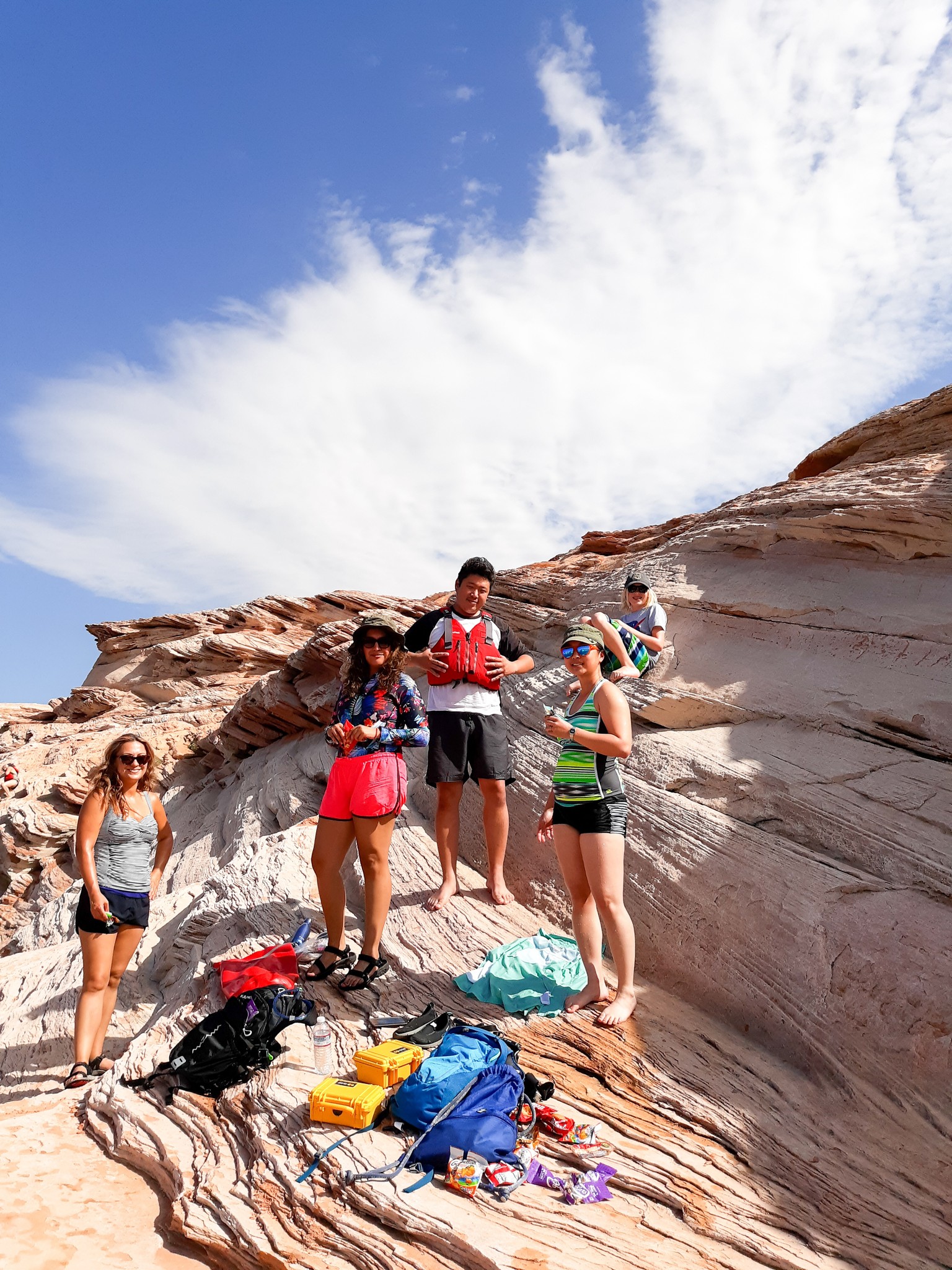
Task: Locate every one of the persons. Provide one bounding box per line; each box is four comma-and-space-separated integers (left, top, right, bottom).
63, 733, 174, 1090
537, 624, 638, 1026
566, 571, 668, 697
0, 764, 19, 800
305, 613, 430, 991
401, 557, 536, 911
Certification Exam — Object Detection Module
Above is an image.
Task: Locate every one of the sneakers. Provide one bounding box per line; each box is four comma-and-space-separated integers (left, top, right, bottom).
409, 1013, 456, 1048
393, 1002, 438, 1039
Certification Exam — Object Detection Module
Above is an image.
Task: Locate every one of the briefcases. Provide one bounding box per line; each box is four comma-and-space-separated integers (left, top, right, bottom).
353, 1039, 424, 1089
308, 1077, 385, 1130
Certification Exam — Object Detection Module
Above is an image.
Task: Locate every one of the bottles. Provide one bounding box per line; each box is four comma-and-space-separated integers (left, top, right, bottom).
313, 1016, 331, 1075
291, 916, 312, 945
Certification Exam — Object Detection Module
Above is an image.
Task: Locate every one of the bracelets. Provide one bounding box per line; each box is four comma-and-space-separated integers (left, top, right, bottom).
374, 728, 378, 740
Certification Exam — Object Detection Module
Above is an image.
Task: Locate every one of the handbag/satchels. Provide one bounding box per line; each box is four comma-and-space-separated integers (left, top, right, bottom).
213, 942, 299, 999
294, 931, 329, 962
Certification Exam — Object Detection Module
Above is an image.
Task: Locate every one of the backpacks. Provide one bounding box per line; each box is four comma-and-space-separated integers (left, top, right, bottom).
169, 985, 319, 1097
390, 1024, 507, 1132
397, 1064, 524, 1173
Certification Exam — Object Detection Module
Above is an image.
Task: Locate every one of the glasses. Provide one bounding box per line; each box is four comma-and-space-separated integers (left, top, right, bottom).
628, 585, 648, 593
115, 755, 151, 765
560, 644, 600, 659
361, 636, 393, 649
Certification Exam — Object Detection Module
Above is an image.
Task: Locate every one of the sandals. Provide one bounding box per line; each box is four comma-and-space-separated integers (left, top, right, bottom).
64, 1062, 92, 1089
338, 953, 389, 991
89, 1053, 114, 1076
305, 946, 356, 981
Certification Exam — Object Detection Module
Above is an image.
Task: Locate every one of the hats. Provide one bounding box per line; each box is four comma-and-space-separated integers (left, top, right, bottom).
347, 614, 409, 659
625, 572, 650, 590
560, 624, 604, 650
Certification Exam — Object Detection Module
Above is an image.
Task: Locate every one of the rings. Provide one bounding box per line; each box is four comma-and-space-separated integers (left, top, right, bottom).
550, 726, 552, 731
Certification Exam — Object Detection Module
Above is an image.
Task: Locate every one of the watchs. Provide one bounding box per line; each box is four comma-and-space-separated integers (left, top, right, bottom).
569, 726, 576, 741
